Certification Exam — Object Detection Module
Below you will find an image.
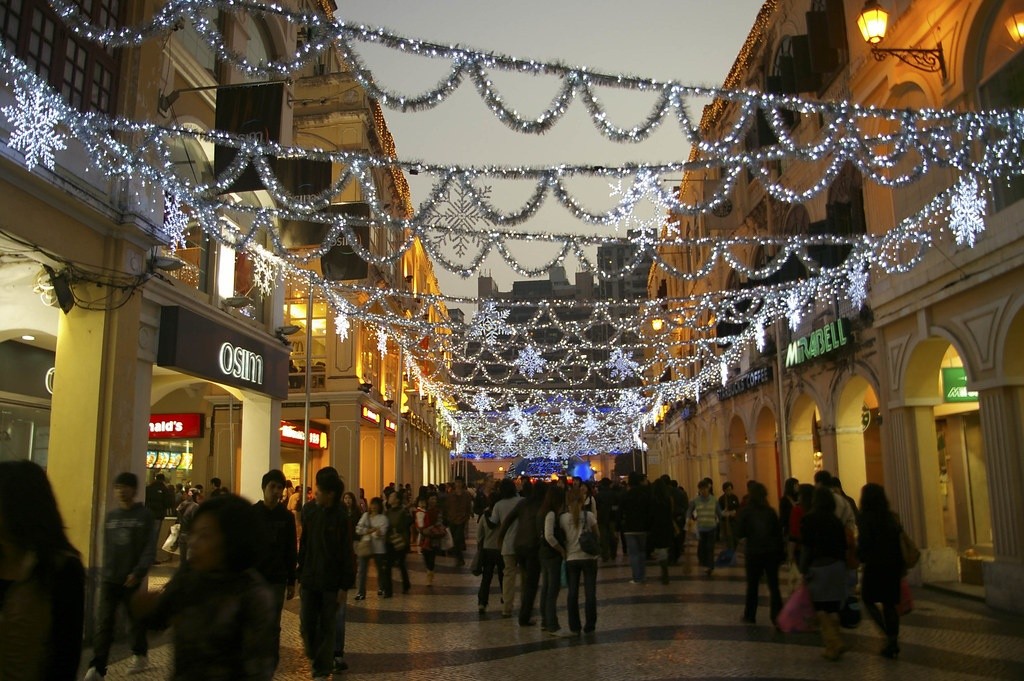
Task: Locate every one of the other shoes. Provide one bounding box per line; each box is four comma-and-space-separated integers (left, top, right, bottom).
569, 631, 580, 636
519, 619, 536, 626
740, 614, 745, 622
822, 635, 848, 661
332, 661, 349, 672
630, 580, 642, 585
312, 671, 330, 680
583, 630, 595, 637
478, 604, 485, 610
425, 568, 433, 586
500, 610, 512, 617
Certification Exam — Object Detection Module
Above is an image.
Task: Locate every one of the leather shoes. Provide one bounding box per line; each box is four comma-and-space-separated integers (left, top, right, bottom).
548, 628, 569, 637
540, 625, 547, 631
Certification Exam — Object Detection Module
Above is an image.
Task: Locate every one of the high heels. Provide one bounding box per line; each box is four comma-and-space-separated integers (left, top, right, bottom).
880, 639, 901, 657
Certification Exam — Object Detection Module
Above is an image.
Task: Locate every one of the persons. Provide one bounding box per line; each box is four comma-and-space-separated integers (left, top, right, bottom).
0, 459, 919, 681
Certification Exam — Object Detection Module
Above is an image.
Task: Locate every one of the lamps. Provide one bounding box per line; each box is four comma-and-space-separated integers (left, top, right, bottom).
154, 256, 187, 271
1003, 7, 1024, 45
384, 399, 394, 408
854, 0, 947, 81
42, 262, 75, 315
424, 312, 428, 319
360, 383, 374, 393
226, 297, 251, 308
278, 325, 302, 335
407, 275, 414, 284
652, 317, 665, 332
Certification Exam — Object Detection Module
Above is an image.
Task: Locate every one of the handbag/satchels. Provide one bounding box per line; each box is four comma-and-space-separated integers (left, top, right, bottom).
353, 539, 373, 557
537, 527, 566, 545
896, 577, 916, 616
898, 526, 921, 568
579, 532, 602, 555
777, 563, 817, 633
471, 536, 484, 576
390, 533, 406, 548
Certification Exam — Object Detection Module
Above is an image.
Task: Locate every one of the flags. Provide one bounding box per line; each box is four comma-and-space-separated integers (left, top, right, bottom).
214, 78, 295, 196
276, 154, 331, 247
320, 204, 371, 279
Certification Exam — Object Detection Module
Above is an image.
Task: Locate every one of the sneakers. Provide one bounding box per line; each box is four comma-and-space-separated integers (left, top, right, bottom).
378, 590, 384, 595
83, 666, 104, 681
353, 592, 366, 600
125, 652, 150, 674
